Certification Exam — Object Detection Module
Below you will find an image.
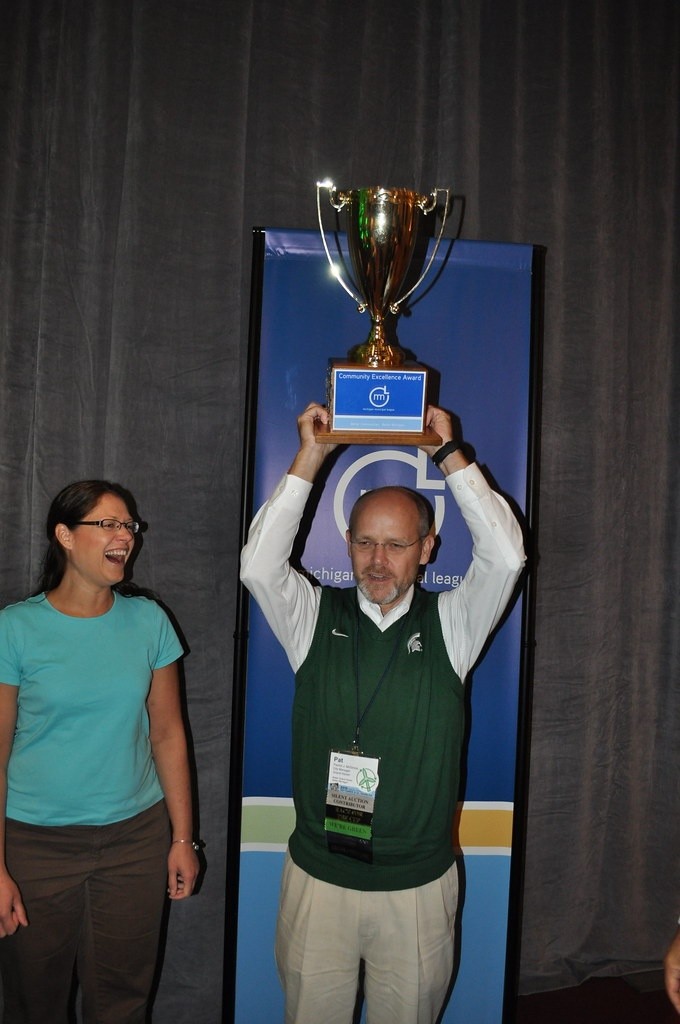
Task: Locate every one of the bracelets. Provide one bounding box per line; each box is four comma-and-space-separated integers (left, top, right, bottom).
430, 436, 460, 467
171, 840, 200, 851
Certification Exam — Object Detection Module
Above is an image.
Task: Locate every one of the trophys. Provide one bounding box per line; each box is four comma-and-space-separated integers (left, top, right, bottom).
312, 177, 450, 448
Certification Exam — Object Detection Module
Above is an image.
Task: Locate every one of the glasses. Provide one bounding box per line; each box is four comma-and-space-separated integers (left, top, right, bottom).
350, 538, 421, 554
66, 519, 140, 534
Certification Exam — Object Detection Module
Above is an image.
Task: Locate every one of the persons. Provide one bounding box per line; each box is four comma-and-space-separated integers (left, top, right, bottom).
1, 479, 201, 1023
666, 917, 680, 1013
238, 403, 527, 1023
